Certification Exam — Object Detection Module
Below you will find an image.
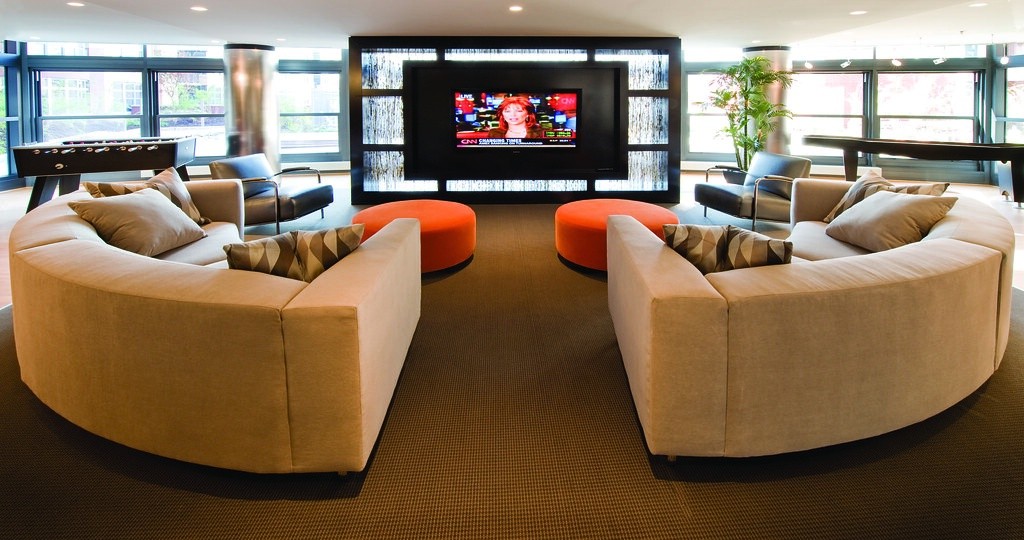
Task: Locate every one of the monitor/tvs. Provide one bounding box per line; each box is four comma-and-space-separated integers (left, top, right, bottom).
451, 88, 582, 148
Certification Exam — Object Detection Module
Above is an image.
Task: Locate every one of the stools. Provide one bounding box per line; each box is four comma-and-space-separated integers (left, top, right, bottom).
350, 198, 477, 273
555, 198, 680, 271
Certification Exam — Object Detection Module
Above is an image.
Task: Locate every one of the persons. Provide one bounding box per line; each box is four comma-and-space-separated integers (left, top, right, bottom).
487, 96, 543, 138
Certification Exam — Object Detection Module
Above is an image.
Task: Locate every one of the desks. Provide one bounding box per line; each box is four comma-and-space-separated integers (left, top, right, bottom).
804, 134, 1024, 208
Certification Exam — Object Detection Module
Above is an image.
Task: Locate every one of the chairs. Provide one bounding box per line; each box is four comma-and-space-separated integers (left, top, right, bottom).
695, 150, 812, 232
208, 153, 334, 235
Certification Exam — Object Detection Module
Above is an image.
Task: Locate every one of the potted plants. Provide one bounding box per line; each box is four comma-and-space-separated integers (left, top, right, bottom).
700, 57, 795, 185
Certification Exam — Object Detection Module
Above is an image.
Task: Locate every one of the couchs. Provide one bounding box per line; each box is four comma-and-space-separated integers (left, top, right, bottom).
606, 178, 1015, 458
10, 179, 421, 475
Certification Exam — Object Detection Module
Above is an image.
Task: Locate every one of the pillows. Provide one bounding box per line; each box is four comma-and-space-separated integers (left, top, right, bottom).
222, 224, 365, 285
664, 223, 794, 276
65, 187, 208, 258
82, 166, 213, 227
825, 189, 958, 252
825, 169, 950, 226
